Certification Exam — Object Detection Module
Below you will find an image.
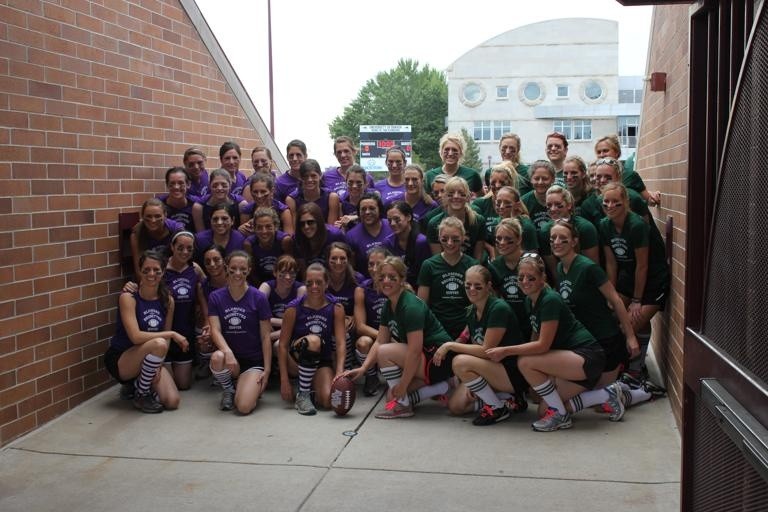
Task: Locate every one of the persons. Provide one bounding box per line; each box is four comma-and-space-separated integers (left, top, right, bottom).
130, 130, 667, 375
104, 250, 189, 413
278, 263, 346, 415
433, 264, 528, 426
485, 253, 625, 431
550, 219, 667, 413
337, 255, 457, 419
122, 232, 210, 389
353, 247, 414, 396
208, 251, 273, 413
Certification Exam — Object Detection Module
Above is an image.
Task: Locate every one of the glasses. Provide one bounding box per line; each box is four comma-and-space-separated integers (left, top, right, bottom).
595, 158, 615, 166
523, 253, 540, 260
552, 217, 568, 224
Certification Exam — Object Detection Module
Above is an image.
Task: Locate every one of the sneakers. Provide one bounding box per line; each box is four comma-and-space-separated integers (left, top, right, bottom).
602, 382, 625, 421
363, 374, 379, 396
532, 407, 573, 431
191, 346, 236, 410
295, 390, 318, 415
473, 403, 510, 425
429, 375, 460, 405
500, 393, 528, 413
119, 378, 164, 413
270, 356, 279, 378
374, 400, 415, 419
618, 365, 666, 401
